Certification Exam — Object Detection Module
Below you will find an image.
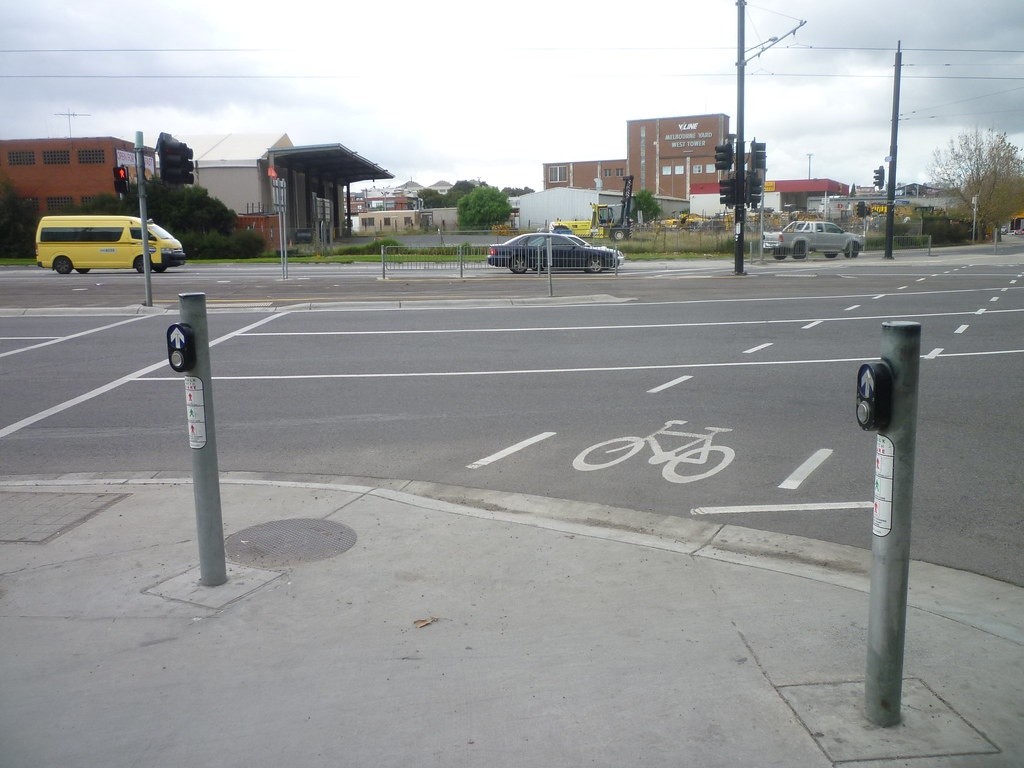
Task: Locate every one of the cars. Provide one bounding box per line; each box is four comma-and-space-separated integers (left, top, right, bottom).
487, 231, 625, 274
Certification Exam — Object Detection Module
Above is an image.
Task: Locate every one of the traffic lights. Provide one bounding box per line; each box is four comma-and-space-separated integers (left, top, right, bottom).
720, 179, 735, 205
113, 166, 130, 193
750, 139, 766, 170
855, 202, 866, 218
746, 175, 763, 204
874, 166, 885, 189
158, 132, 195, 185
714, 142, 732, 171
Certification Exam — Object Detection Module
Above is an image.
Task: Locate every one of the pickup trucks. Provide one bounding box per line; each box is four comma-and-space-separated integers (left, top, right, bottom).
761, 220, 864, 260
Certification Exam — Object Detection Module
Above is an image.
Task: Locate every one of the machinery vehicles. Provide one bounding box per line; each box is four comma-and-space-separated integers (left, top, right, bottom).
550, 175, 634, 244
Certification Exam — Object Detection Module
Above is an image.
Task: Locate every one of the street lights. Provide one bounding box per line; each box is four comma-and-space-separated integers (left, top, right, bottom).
732, 36, 778, 275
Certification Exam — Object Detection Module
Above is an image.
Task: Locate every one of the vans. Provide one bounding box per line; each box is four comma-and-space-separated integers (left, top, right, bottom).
35, 214, 187, 275
